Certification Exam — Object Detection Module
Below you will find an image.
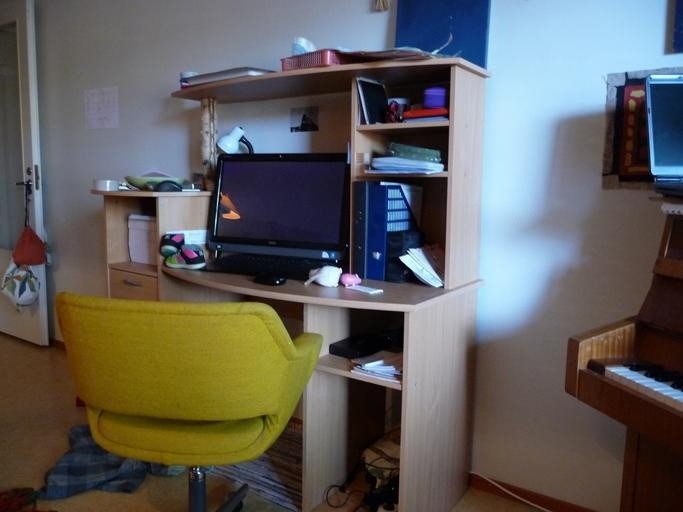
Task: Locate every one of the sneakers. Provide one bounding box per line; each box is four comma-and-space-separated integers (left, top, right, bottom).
165, 243, 206, 270
159, 233, 184, 256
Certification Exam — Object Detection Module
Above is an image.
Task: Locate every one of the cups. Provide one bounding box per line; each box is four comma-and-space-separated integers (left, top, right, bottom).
386, 97, 409, 123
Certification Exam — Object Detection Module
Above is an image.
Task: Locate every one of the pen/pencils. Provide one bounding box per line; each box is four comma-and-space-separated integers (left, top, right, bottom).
365, 358, 385, 367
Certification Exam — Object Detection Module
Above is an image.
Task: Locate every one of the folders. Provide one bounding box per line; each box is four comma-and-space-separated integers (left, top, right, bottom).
353, 180, 417, 281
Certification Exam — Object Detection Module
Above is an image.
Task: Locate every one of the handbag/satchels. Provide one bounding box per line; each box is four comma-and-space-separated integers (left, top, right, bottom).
12, 226, 44, 266
2, 261, 39, 310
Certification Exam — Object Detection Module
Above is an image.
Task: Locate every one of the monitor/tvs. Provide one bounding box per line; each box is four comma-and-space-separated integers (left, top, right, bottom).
645, 74, 683, 177
201, 151, 350, 281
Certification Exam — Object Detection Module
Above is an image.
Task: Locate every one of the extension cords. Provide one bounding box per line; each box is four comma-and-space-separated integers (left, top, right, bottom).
377, 502, 398, 512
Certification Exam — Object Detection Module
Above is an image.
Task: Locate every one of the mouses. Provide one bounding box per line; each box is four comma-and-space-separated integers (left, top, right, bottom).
156, 180, 182, 192
254, 268, 289, 285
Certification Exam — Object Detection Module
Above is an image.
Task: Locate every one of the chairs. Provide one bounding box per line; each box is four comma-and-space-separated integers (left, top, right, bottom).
54, 289, 323, 512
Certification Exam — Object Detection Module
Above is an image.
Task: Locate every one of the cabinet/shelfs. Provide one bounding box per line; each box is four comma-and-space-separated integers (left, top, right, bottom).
303, 290, 481, 511
348, 65, 484, 288
104, 195, 242, 301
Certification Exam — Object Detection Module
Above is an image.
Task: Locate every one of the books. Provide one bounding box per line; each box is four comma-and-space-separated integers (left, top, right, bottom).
356, 76, 450, 176
184, 67, 276, 91
350, 352, 403, 383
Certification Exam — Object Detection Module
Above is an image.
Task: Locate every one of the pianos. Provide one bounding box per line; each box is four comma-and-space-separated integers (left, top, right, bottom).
565, 197, 683, 512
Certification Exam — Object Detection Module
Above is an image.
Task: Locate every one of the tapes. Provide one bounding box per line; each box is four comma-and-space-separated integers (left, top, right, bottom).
91, 179, 119, 192
